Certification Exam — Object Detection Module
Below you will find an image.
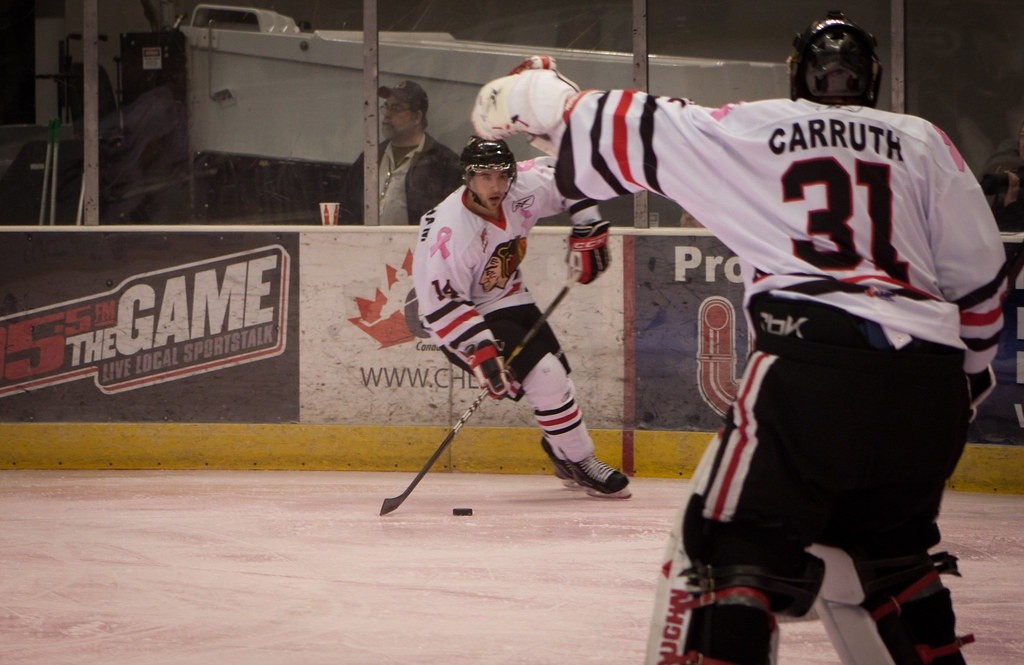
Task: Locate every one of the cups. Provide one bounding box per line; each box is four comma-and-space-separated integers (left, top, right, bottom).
320, 202, 340, 225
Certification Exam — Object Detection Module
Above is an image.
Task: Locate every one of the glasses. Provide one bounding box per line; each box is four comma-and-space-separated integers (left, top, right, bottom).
380, 105, 412, 114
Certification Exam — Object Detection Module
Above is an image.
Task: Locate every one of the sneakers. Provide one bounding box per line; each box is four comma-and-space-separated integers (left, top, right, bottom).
540, 435, 583, 489
565, 452, 630, 498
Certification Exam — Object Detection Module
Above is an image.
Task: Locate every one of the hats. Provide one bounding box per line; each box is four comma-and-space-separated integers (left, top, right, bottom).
376, 80, 429, 111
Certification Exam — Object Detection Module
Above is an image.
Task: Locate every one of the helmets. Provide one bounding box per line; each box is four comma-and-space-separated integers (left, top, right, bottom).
460, 136, 518, 178
787, 10, 883, 109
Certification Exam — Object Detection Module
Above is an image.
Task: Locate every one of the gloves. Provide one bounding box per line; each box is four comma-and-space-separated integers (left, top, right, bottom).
470, 59, 580, 142
563, 219, 612, 284
469, 337, 526, 403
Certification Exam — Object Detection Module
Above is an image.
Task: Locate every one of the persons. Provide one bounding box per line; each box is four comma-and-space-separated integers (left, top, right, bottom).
339, 82, 464, 226
992, 123, 1024, 231
415, 137, 632, 498
470, 17, 1010, 665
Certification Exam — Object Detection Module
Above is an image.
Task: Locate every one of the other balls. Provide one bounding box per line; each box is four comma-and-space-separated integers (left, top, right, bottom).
451, 506, 475, 517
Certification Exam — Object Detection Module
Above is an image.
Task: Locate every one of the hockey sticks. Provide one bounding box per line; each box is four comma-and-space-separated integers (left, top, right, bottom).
378, 270, 584, 517
